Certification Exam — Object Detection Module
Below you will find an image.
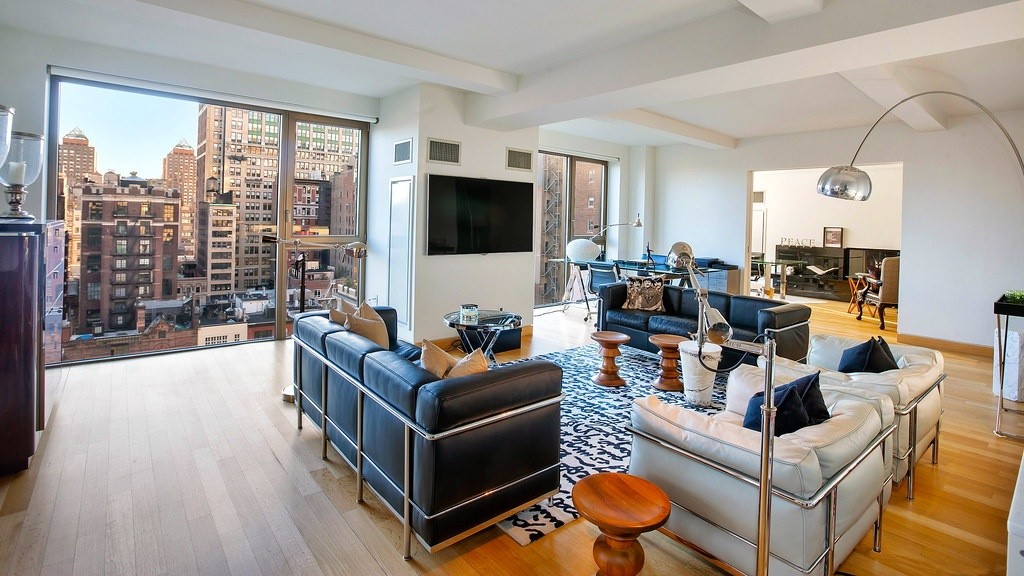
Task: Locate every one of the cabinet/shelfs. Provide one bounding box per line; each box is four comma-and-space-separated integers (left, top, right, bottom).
0, 220, 66, 475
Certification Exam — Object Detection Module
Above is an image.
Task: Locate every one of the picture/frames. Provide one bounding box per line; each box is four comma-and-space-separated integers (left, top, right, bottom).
823, 227, 843, 249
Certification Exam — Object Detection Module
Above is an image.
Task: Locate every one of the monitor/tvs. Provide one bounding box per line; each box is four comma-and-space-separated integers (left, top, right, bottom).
426, 173, 535, 256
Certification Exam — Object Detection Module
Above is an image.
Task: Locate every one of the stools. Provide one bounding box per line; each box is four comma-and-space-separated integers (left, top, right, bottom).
572, 474, 671, 576
591, 331, 631, 386
648, 334, 691, 391
458, 326, 521, 355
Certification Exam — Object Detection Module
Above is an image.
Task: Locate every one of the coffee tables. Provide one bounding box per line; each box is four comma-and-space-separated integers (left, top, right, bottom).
846, 275, 886, 317
751, 259, 808, 297
444, 310, 522, 368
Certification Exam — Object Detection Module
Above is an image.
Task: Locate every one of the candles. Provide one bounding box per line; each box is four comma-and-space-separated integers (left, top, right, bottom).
8, 160, 26, 184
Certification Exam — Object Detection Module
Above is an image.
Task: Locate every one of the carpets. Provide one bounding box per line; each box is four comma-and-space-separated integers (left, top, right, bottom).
486, 341, 731, 548
773, 276, 864, 303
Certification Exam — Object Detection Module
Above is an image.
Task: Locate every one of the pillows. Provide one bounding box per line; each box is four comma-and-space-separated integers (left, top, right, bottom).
837, 336, 899, 375
622, 274, 666, 311
329, 299, 359, 324
345, 301, 389, 349
744, 370, 831, 436
421, 338, 488, 380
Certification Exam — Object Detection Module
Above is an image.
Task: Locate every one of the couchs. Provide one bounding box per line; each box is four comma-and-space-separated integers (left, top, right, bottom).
758, 335, 947, 499
596, 281, 811, 372
628, 366, 898, 576
292, 306, 563, 561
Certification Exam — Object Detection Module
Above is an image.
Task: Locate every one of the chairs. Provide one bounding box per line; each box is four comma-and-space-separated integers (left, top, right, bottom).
585, 260, 623, 294
857, 255, 900, 330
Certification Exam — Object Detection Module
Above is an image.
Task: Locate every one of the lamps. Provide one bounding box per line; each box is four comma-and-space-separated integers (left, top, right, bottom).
590, 218, 643, 242
816, 90, 1024, 201
263, 235, 368, 402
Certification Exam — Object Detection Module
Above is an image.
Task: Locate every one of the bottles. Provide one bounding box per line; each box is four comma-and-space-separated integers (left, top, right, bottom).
460, 303, 478, 326
